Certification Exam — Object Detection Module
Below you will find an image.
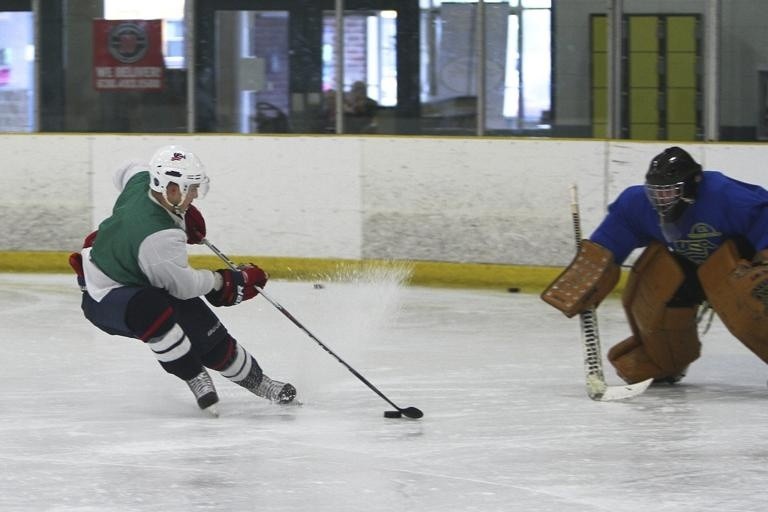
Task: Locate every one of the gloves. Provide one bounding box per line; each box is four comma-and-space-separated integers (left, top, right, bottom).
206, 264, 268, 308
187, 204, 206, 244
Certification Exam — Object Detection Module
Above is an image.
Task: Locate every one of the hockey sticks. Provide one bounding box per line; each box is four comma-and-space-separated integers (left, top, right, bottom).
194, 230, 424, 418
568, 183, 655, 402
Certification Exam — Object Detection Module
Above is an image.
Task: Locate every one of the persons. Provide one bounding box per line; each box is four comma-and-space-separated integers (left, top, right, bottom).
70, 146, 296, 410
541, 146, 768, 387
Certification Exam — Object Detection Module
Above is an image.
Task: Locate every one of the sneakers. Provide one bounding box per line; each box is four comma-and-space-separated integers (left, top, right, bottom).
186, 365, 219, 411
256, 376, 297, 405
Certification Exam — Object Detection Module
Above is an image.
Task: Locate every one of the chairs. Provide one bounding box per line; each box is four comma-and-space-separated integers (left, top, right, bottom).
257, 103, 286, 133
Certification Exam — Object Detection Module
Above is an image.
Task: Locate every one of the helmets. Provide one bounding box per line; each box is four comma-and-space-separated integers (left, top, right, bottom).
644, 146, 703, 222
149, 144, 211, 209
